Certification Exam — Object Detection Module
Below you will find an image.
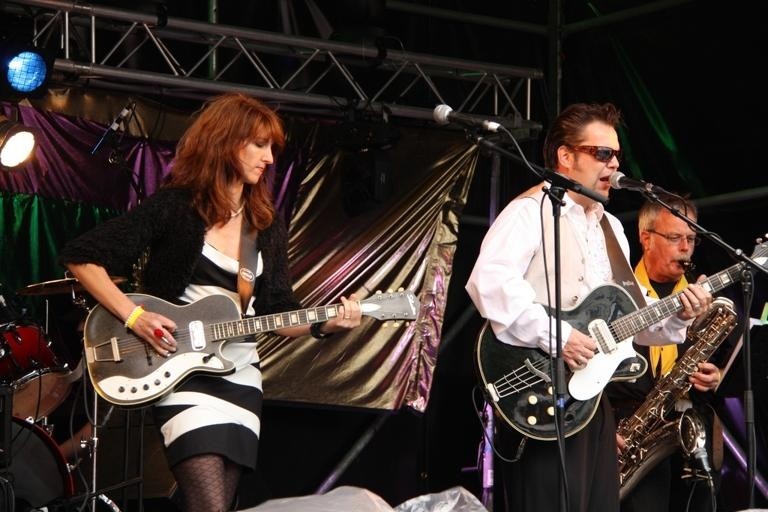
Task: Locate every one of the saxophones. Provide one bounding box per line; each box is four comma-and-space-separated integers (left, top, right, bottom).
617, 258, 737, 501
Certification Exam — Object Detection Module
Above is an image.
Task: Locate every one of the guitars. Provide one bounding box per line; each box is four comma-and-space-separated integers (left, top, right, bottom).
83, 288, 418, 405
473, 234, 767, 441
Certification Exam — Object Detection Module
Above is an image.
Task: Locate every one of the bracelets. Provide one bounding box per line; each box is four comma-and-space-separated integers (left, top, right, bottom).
125, 305, 143, 330
128, 309, 145, 329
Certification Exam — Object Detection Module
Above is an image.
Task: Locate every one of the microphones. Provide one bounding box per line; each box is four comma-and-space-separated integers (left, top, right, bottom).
609, 172, 664, 194
432, 104, 500, 133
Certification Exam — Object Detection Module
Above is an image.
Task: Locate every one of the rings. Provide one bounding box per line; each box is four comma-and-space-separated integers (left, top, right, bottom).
152, 328, 165, 339
693, 302, 705, 311
344, 315, 349, 320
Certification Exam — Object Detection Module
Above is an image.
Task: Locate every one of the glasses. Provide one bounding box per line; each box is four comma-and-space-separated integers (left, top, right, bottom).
648, 230, 701, 247
569, 146, 623, 162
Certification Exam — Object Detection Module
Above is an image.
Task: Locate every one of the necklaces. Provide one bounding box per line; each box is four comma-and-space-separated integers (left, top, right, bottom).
225, 197, 245, 218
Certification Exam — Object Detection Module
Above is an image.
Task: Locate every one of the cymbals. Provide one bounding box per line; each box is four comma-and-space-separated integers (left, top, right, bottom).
21, 278, 126, 293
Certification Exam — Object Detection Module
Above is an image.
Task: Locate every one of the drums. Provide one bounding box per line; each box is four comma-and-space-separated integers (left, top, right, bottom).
1, 324, 70, 422
0, 417, 74, 511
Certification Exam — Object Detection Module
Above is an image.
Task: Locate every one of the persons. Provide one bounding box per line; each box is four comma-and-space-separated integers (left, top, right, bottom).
464, 102, 713, 512
604, 194, 723, 511
63, 92, 362, 511
53, 376, 113, 487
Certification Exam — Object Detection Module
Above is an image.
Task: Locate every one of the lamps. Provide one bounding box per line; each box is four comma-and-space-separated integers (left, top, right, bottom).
0, 113, 38, 171
0, 45, 51, 100
